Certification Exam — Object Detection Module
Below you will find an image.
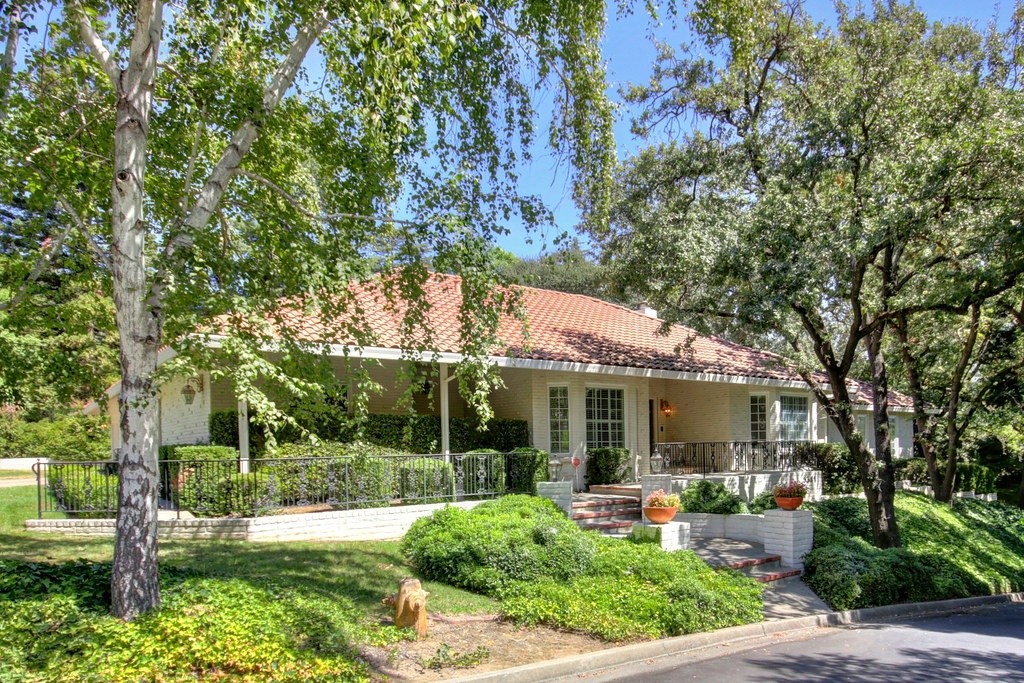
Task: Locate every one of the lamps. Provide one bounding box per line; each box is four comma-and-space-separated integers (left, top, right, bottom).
661, 401, 672, 417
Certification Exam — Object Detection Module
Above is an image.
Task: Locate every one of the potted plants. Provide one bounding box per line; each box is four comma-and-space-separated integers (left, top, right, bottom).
641, 489, 680, 525
773, 480, 806, 511
